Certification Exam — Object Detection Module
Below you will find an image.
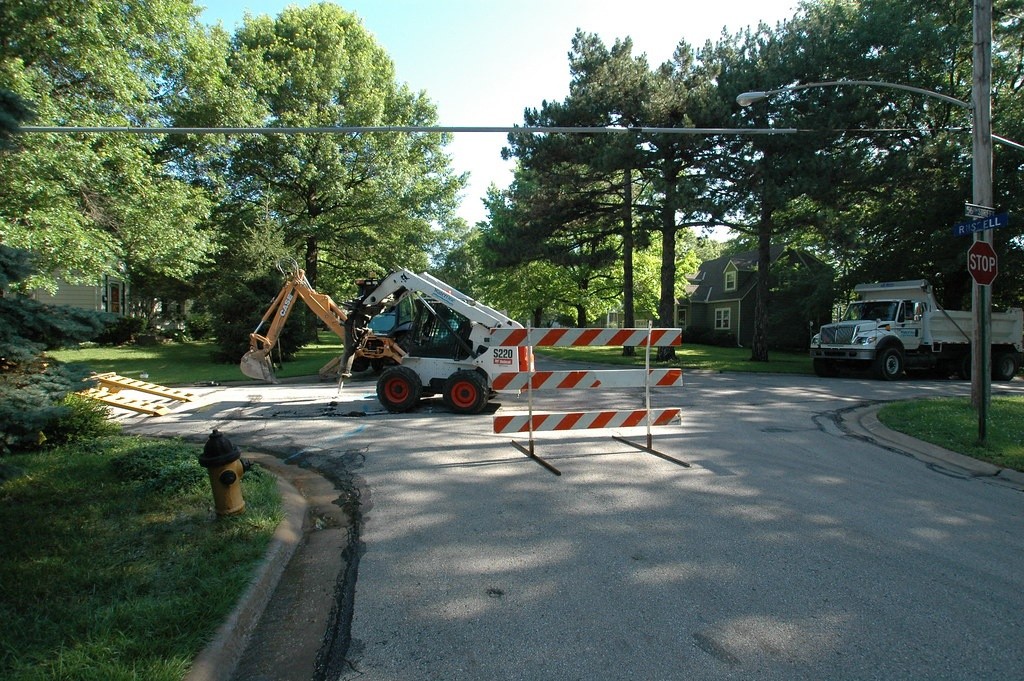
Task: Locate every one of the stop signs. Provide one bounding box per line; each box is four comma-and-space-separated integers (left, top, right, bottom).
967, 240, 998, 286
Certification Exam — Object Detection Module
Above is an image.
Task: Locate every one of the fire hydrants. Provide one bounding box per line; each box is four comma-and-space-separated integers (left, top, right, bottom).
198, 429, 254, 519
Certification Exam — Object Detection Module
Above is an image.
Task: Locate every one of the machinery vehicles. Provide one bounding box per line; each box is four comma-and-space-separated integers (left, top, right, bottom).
240, 256, 534, 415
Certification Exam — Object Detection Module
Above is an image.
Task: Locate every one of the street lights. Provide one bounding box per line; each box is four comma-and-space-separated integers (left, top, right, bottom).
736, 0, 992, 447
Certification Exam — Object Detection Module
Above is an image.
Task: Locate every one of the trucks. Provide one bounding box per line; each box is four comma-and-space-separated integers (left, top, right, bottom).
810, 279, 1024, 381
